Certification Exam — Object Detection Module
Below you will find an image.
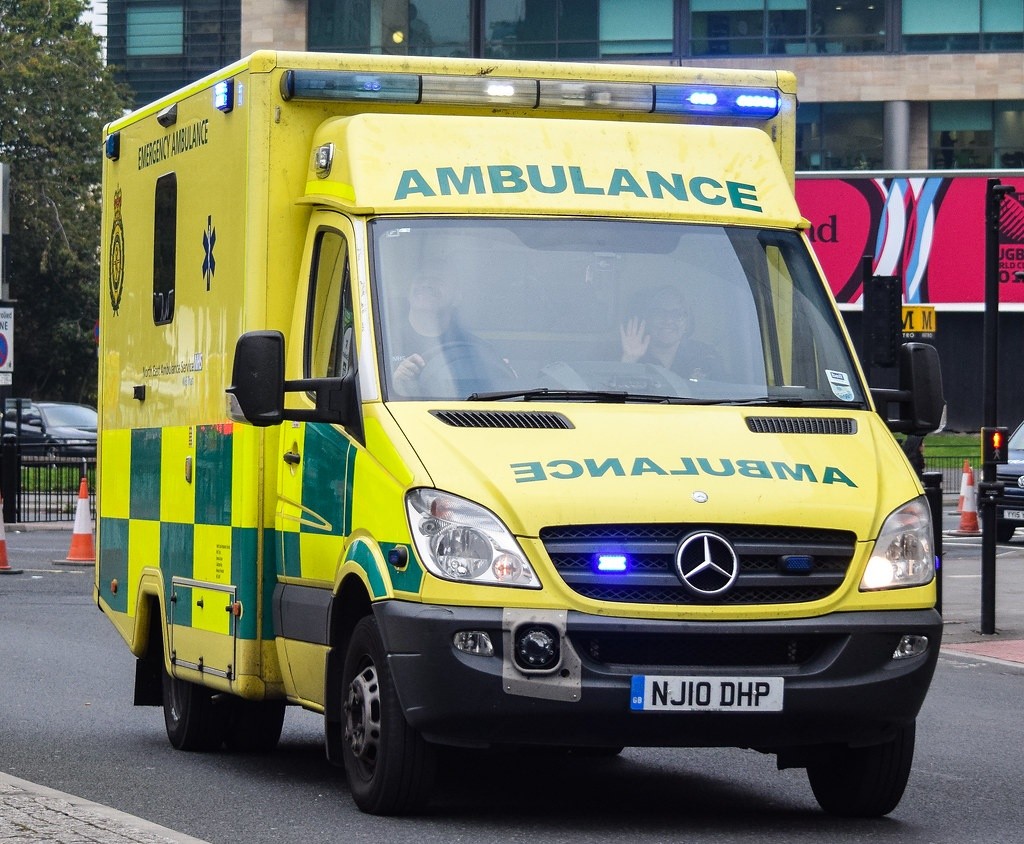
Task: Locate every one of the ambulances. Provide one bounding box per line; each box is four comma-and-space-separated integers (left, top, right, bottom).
89, 45, 943, 823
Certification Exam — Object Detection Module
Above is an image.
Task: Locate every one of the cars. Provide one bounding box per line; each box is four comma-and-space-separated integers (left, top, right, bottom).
978, 419, 1024, 545
0, 397, 101, 463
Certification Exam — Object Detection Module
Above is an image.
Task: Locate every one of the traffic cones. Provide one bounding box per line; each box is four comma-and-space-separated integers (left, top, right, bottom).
52, 477, 95, 566
949, 468, 983, 537
0, 490, 24, 577
947, 460, 971, 516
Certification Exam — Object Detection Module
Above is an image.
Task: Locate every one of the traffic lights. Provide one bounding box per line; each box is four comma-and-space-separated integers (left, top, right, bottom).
978, 426, 1009, 469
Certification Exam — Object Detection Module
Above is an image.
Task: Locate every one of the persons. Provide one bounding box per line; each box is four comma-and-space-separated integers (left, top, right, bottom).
812, 12, 828, 53
940, 131, 955, 168
615, 290, 730, 381
904, 435, 925, 481
389, 271, 520, 389
